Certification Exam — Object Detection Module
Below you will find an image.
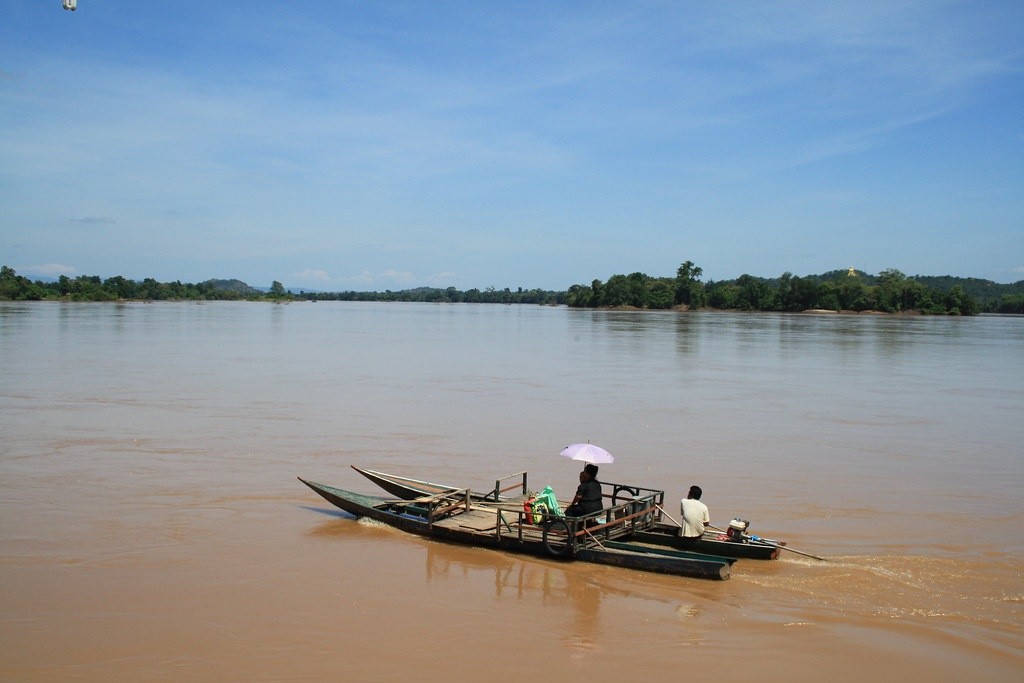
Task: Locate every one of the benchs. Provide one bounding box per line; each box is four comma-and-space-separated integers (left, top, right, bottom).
567, 504, 625, 544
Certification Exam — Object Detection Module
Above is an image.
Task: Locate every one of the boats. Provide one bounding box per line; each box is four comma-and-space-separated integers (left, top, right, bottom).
296, 465, 787, 580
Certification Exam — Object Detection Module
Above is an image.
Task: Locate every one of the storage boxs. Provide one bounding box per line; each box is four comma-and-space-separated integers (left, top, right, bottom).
627, 495, 653, 522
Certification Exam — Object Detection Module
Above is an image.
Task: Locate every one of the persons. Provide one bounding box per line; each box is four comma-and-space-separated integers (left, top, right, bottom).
681, 486, 709, 539
565, 464, 602, 530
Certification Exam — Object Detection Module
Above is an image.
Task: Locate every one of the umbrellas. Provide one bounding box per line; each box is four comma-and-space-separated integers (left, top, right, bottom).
560, 440, 615, 465
